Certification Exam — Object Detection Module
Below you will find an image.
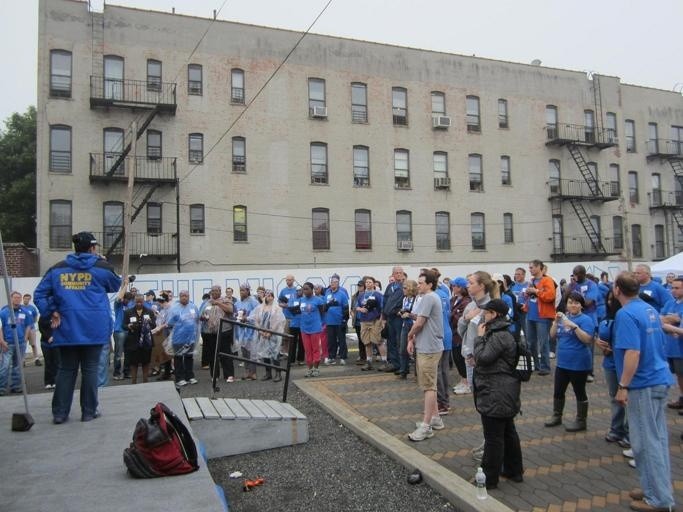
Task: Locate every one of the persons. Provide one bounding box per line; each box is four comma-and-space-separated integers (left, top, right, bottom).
469, 296, 528, 490
113, 282, 200, 385
351, 267, 418, 380
197, 285, 287, 382
1, 290, 56, 394
606, 271, 677, 511
279, 274, 350, 364
455, 260, 567, 464
545, 265, 683, 467
398, 269, 473, 441
33, 230, 126, 426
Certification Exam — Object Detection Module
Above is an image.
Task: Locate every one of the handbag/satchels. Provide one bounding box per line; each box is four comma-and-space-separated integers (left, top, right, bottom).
512, 341, 536, 383
120, 402, 199, 477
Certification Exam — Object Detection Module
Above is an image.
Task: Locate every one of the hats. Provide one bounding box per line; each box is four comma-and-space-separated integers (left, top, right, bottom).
69, 231, 102, 249
474, 299, 508, 315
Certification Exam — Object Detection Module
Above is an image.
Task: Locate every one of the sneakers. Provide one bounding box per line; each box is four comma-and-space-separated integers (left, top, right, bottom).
451, 381, 473, 394
538, 366, 550, 375
406, 402, 451, 440
355, 355, 407, 381
290, 356, 345, 378
604, 427, 661, 509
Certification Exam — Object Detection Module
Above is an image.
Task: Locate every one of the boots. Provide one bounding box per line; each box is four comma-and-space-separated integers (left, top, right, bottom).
563, 400, 588, 431
543, 396, 565, 427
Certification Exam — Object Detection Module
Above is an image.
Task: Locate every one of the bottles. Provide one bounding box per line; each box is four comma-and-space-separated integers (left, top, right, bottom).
475, 466, 487, 499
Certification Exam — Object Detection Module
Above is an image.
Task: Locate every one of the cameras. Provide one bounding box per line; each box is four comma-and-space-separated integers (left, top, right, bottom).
400, 308, 410, 314
529, 284, 536, 298
128, 276, 136, 283
